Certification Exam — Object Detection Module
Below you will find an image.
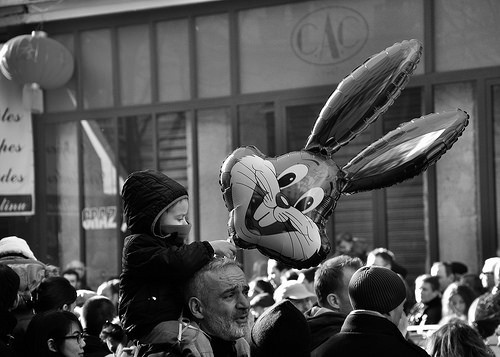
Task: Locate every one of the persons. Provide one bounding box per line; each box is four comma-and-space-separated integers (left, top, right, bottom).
118, 169, 250, 357
0, 235, 500, 357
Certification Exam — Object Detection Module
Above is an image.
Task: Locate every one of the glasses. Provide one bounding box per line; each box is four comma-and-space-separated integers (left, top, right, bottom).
53, 332, 87, 344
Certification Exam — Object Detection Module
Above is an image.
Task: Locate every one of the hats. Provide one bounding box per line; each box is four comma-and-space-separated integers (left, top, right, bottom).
348, 266, 406, 315
273, 280, 317, 304
34, 277, 77, 312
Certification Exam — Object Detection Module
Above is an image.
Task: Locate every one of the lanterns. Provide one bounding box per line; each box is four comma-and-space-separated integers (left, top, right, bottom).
0, 31, 74, 114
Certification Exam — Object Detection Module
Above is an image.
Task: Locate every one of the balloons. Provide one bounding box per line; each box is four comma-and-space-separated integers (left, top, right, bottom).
218, 39, 470, 270
